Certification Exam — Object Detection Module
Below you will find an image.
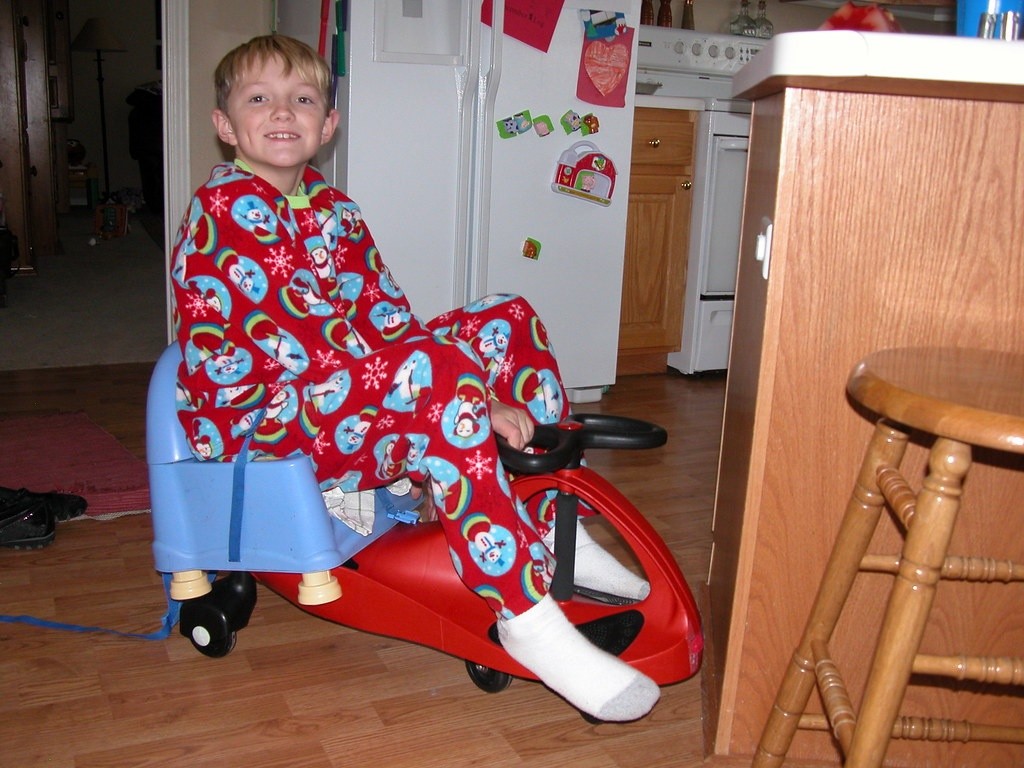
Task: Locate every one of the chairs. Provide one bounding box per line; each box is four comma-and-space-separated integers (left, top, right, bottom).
145, 337, 427, 606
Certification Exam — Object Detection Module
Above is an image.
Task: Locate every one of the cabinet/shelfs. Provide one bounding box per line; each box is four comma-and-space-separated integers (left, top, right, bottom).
702, 75, 1024, 758
615, 107, 703, 377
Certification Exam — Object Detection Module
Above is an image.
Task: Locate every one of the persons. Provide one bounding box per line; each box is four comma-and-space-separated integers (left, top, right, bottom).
169, 35, 663, 722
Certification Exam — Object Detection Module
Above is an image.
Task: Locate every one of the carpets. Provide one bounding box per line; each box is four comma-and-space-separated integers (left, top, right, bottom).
0, 207, 164, 371
0, 413, 154, 522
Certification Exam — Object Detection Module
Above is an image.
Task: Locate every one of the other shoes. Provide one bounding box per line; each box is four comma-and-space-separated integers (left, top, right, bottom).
0, 486, 87, 521
0, 501, 55, 549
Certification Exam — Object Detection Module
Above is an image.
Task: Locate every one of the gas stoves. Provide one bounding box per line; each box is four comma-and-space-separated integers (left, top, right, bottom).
634, 23, 782, 112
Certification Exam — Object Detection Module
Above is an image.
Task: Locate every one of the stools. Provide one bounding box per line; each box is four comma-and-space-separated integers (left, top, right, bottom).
752, 347, 1024, 768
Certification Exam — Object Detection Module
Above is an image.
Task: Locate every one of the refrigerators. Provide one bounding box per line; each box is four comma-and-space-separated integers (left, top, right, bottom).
271, 0, 641, 404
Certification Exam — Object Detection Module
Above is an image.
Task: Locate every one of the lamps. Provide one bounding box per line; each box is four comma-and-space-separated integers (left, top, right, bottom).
70, 17, 128, 202
